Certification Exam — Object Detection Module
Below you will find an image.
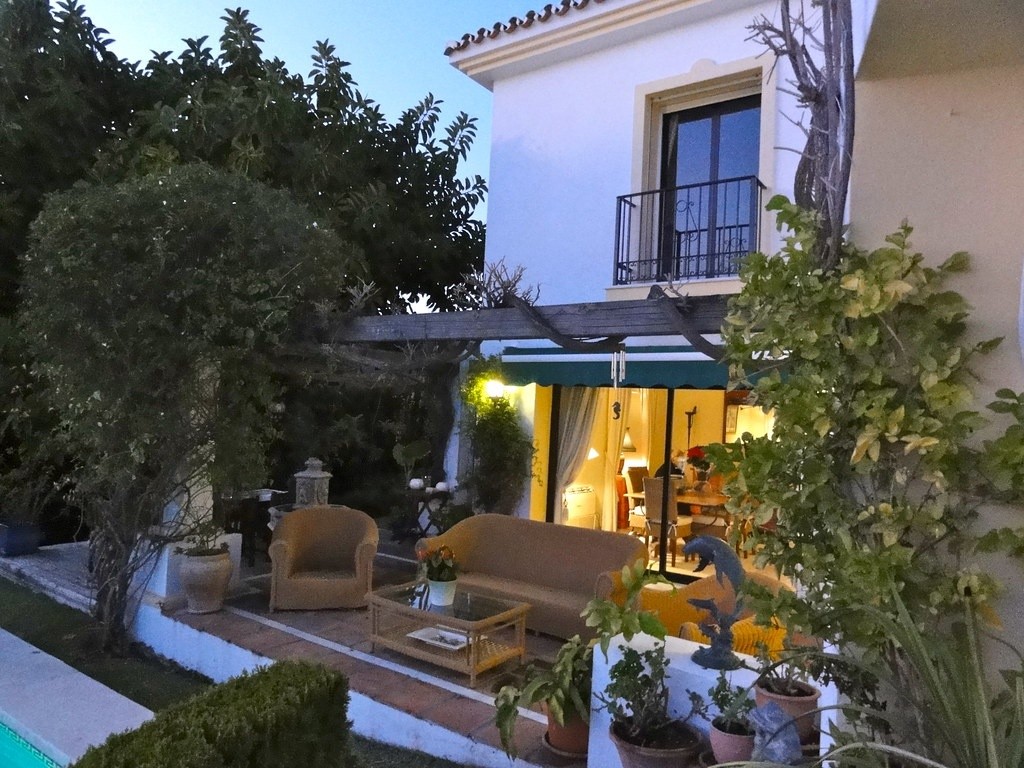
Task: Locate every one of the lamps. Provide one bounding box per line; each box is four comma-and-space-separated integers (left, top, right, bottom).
617, 427, 637, 475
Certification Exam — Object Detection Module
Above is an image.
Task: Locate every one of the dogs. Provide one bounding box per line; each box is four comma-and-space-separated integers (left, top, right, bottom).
653, 448, 689, 478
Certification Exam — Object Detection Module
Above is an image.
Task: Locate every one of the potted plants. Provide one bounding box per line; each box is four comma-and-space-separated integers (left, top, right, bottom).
752, 638, 821, 742
172, 520, 233, 615
490, 631, 599, 763
707, 669, 755, 763
590, 640, 704, 768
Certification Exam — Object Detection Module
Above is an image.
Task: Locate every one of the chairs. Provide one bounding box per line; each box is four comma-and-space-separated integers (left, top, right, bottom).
627, 465, 649, 536
641, 475, 695, 567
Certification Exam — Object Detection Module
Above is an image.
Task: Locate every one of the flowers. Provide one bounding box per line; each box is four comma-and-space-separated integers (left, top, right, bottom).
416, 544, 462, 581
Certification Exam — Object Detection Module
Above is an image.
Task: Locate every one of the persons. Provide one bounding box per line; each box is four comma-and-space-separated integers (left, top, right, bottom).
652, 449, 688, 479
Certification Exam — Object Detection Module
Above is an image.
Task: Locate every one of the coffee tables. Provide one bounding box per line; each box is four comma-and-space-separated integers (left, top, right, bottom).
364, 580, 533, 689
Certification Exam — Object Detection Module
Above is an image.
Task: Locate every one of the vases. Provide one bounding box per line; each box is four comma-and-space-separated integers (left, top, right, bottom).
425, 577, 457, 606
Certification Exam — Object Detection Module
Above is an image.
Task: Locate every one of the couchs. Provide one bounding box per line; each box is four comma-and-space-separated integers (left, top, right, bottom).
267, 504, 379, 614
610, 568, 792, 664
414, 513, 648, 647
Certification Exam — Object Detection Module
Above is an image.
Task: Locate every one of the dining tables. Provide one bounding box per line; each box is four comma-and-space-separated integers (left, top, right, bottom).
623, 489, 748, 559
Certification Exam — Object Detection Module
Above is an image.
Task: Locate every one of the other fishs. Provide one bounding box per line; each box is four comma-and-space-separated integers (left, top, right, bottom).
683, 535, 750, 621
